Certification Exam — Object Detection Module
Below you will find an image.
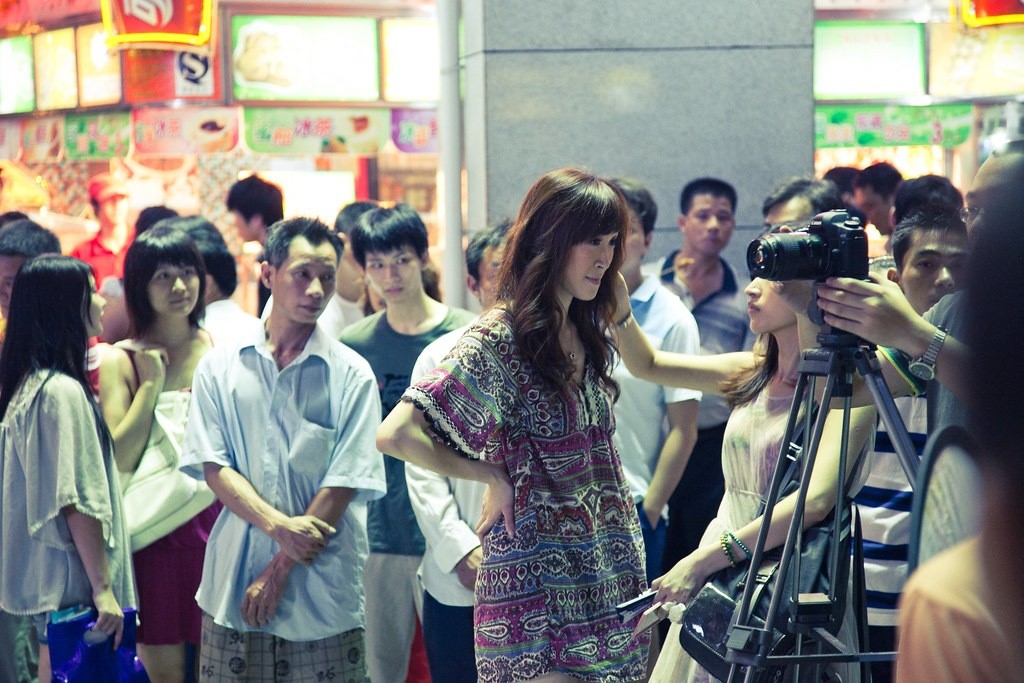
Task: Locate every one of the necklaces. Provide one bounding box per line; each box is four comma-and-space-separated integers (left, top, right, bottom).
558, 320, 576, 361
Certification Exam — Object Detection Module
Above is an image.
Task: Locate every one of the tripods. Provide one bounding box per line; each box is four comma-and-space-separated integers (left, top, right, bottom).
726, 282, 923, 683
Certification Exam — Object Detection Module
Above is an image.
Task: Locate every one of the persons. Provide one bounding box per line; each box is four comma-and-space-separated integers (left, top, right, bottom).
604, 179, 702, 588
640, 176, 758, 578
377, 169, 651, 683
764, 162, 1023, 683
602, 217, 875, 683
0, 175, 516, 683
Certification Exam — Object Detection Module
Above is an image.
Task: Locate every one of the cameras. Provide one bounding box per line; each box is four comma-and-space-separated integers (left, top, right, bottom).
746, 209, 870, 282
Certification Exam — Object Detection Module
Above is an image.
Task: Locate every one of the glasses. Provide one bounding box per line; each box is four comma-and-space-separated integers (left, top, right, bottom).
958, 202, 992, 223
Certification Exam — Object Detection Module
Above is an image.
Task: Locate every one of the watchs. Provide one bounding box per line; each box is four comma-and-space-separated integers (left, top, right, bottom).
909, 325, 949, 381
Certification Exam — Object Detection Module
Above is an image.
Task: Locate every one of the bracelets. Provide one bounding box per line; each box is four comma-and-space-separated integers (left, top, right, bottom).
608, 311, 635, 332
720, 529, 752, 568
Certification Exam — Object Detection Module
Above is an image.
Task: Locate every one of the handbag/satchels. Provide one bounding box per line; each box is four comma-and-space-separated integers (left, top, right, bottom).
679, 390, 883, 683
114, 389, 216, 552
47, 606, 152, 683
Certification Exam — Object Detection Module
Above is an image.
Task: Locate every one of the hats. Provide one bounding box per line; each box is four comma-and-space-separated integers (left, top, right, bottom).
89, 173, 127, 200
